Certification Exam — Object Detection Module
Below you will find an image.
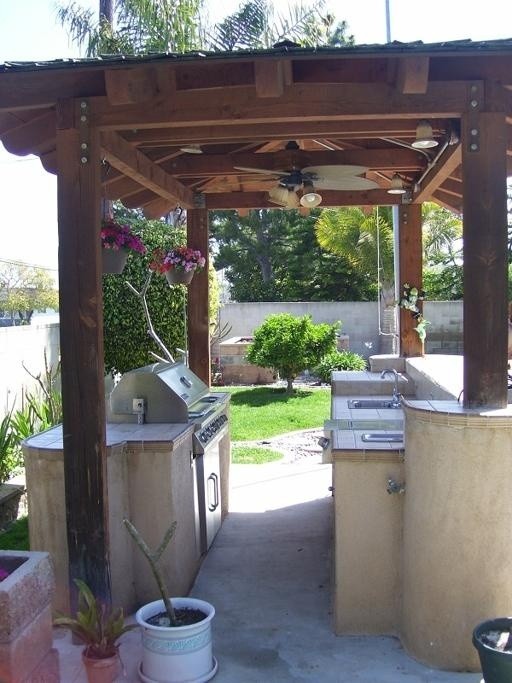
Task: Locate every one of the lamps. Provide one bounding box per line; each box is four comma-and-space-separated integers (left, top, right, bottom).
267, 175, 324, 212
179, 142, 206, 154
383, 171, 408, 196
410, 118, 440, 151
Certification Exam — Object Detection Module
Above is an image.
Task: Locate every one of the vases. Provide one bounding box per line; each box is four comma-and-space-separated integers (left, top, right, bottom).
471, 614, 512, 682
164, 266, 198, 286
99, 245, 133, 275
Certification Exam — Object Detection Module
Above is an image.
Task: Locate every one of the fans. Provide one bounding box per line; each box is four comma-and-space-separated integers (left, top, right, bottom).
221, 141, 379, 214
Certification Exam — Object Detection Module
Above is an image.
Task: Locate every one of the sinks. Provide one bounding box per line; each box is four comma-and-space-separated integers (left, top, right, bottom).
347, 399, 401, 409
363, 433, 404, 442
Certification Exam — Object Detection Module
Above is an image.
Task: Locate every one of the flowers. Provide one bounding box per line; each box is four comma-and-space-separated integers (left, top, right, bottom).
160, 245, 209, 272
98, 219, 151, 258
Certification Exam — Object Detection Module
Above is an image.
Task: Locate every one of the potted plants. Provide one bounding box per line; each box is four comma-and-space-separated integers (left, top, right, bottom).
48, 576, 141, 682
119, 516, 220, 682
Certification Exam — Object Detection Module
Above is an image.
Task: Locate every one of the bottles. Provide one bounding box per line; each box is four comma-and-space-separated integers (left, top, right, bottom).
138, 405, 143, 425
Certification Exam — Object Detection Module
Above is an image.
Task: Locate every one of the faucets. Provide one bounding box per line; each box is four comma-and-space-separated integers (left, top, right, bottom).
380, 368, 402, 408
